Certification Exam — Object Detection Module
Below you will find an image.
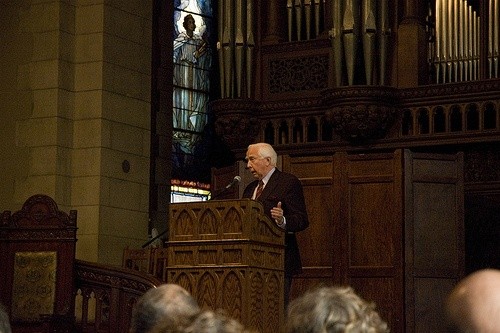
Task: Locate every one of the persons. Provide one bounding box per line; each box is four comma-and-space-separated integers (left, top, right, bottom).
242, 143, 309, 311
0, 304, 11, 333
75, 288, 84, 321
185, 310, 249, 333
446, 269, 500, 333
88, 292, 95, 323
286, 287, 390, 333
129, 284, 200, 333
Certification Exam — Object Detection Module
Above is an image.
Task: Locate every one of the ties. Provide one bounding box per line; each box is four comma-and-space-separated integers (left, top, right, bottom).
255, 180, 264, 200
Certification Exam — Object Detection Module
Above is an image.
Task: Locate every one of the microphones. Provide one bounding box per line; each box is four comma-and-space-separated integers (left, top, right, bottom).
226, 176, 242, 190
